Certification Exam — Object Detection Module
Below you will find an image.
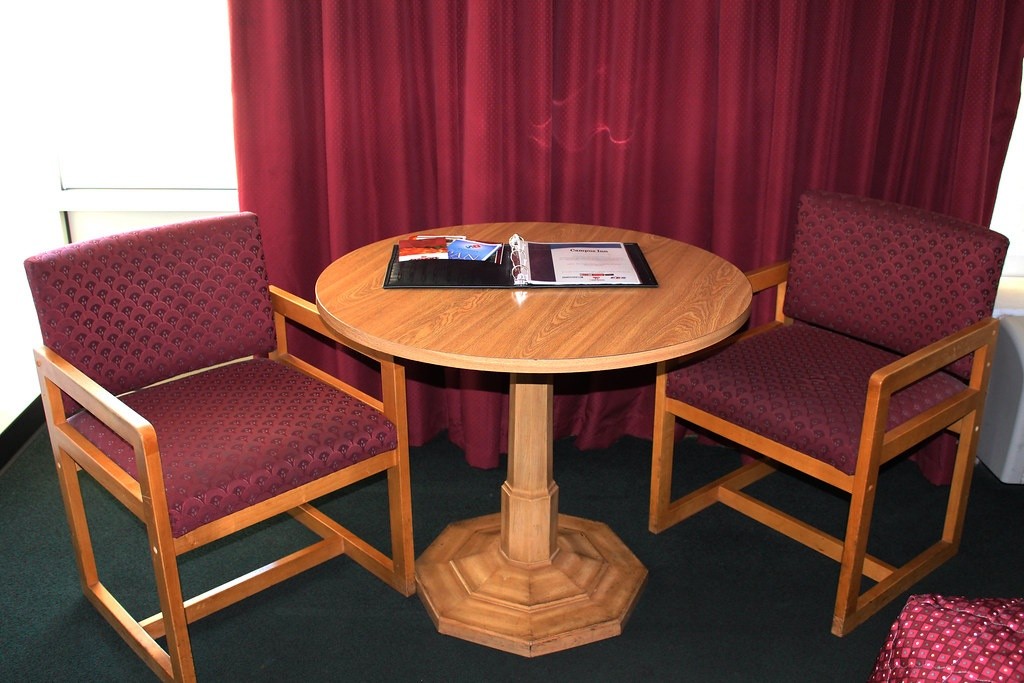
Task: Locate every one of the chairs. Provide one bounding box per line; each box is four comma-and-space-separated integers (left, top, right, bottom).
24, 211, 418, 683
648, 187, 1010, 639
867, 594, 1024, 683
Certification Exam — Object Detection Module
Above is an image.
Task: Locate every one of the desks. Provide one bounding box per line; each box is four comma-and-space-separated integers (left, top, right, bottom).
315, 223, 753, 659
